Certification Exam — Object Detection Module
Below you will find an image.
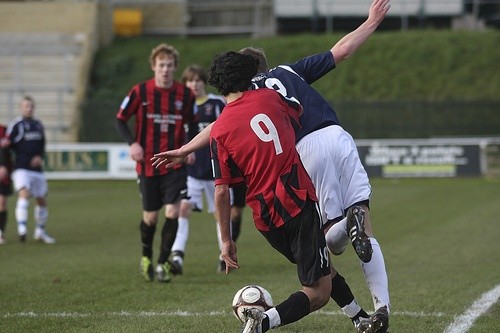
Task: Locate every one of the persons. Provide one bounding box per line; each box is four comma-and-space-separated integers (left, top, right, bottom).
208, 52, 390, 333
115, 44, 198, 282
148, 0, 392, 333
228, 159, 246, 243
0, 96, 57, 246
167, 65, 232, 274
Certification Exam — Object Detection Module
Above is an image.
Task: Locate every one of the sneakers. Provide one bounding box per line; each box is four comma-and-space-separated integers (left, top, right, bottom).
353, 317, 372, 333
372, 305, 389, 333
241, 307, 269, 333
155, 264, 171, 282
347, 206, 373, 263
0, 230, 7, 245
139, 256, 154, 282
33, 224, 56, 243
17, 221, 27, 235
165, 250, 185, 276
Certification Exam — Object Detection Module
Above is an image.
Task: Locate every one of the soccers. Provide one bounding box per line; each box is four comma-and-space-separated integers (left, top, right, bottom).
232, 284, 273, 324
